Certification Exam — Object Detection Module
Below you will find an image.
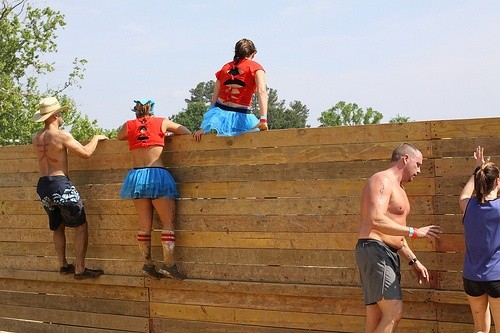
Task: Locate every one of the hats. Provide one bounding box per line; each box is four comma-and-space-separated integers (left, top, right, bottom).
33, 97, 69, 123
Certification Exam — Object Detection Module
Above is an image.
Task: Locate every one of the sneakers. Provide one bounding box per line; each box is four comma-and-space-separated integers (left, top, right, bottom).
159, 264, 186, 280
140, 263, 165, 280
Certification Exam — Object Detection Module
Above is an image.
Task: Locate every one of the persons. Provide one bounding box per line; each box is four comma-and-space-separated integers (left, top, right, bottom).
352, 142, 442, 332
193, 38, 269, 141
119, 97, 192, 279
31, 97, 110, 279
458, 144, 500, 333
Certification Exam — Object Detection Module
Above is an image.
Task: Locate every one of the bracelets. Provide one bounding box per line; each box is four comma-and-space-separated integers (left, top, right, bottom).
408, 257, 418, 265
408, 226, 418, 238
260, 112, 266, 123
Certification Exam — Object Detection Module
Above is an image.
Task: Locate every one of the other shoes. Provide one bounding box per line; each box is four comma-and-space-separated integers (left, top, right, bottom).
60, 264, 75, 275
74, 268, 104, 280
